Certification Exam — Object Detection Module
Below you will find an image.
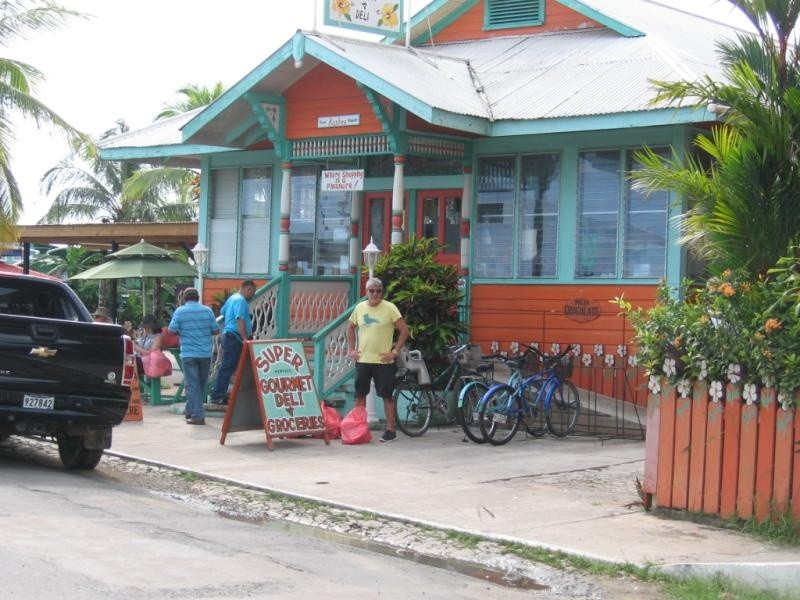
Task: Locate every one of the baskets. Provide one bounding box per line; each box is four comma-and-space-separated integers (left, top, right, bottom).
511, 351, 540, 377
544, 358, 572, 378
448, 345, 482, 369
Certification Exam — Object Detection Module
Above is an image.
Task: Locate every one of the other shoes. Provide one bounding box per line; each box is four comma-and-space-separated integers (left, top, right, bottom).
211, 396, 228, 404
187, 418, 204, 425
185, 413, 191, 419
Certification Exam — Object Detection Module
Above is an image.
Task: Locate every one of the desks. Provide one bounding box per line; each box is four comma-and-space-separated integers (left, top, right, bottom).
165, 348, 208, 403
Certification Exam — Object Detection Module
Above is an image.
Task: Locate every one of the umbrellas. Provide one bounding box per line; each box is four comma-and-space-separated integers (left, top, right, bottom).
68, 238, 199, 319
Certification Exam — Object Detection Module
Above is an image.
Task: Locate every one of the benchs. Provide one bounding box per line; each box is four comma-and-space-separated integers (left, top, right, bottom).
140, 373, 161, 406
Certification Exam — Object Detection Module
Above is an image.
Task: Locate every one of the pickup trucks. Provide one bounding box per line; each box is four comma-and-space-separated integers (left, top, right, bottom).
0, 272, 135, 471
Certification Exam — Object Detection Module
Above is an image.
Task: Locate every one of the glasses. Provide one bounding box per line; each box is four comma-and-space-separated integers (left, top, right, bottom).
368, 289, 382, 293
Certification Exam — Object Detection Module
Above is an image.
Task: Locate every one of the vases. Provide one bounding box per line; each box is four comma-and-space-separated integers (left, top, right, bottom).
635, 371, 800, 534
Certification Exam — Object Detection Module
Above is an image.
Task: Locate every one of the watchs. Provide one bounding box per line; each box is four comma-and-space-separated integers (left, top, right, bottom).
391, 349, 399, 356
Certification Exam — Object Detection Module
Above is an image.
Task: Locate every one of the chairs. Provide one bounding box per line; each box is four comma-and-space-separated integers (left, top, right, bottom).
9, 290, 52, 315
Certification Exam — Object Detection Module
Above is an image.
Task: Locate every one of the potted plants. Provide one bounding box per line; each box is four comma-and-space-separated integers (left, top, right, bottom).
366, 234, 467, 424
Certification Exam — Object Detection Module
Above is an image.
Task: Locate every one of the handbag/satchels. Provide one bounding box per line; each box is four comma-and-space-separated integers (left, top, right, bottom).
160, 327, 179, 348
141, 349, 172, 378
311, 401, 342, 439
341, 406, 372, 445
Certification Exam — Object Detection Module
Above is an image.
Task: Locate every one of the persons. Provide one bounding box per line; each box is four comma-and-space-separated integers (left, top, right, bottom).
347, 278, 409, 441
168, 286, 220, 425
210, 281, 256, 406
93, 307, 162, 374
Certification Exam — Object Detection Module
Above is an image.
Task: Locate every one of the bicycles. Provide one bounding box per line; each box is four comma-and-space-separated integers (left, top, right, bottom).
458, 349, 551, 444
477, 344, 580, 446
394, 343, 478, 436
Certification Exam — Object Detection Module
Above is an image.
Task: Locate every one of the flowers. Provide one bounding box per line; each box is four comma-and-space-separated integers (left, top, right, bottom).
606, 0, 800, 412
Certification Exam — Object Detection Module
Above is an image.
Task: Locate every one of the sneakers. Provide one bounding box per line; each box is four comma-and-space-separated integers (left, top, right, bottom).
380, 430, 398, 442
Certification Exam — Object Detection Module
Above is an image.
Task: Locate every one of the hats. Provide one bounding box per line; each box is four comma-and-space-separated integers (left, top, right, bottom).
184, 288, 198, 297
142, 315, 156, 328
92, 307, 111, 319
